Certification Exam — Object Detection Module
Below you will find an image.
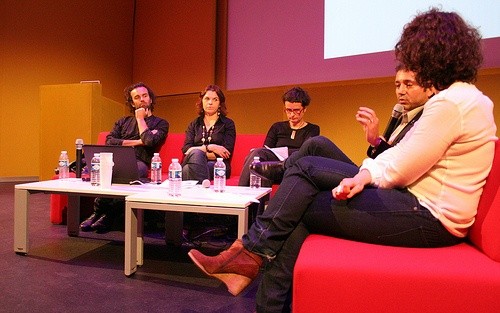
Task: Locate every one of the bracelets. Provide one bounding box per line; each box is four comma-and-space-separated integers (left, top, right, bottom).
206, 144, 212, 153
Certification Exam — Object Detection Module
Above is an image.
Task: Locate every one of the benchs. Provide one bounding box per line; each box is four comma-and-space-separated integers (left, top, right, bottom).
50, 131, 500, 313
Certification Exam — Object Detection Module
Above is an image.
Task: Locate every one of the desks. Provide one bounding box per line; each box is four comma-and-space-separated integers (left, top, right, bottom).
13, 178, 175, 267
124, 184, 273, 275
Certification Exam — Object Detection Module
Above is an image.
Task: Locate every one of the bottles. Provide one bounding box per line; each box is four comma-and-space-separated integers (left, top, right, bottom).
90, 153, 100, 186
151, 153, 162, 185
249, 157, 261, 192
59, 151, 69, 180
168, 158, 182, 198
213, 158, 226, 193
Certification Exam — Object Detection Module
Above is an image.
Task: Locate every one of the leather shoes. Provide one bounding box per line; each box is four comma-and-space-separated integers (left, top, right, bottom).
90, 212, 113, 231
249, 161, 284, 184
80, 213, 99, 231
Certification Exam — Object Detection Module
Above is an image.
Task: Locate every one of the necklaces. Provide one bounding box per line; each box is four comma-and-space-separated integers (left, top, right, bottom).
202, 126, 214, 144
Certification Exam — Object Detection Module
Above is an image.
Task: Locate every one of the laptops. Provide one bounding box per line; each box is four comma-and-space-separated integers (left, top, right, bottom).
82, 145, 151, 184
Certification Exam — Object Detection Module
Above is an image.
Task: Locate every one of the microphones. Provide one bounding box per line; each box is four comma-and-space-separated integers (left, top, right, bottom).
383, 104, 404, 143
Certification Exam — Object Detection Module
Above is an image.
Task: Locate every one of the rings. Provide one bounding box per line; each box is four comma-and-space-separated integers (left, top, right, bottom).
336, 186, 341, 194
224, 152, 226, 153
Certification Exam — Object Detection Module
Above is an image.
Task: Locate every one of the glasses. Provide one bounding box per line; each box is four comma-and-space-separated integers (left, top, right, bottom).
284, 107, 305, 114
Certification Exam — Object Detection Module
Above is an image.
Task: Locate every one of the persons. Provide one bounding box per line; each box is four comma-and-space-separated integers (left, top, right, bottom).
229, 87, 320, 237
182, 85, 235, 238
81, 82, 169, 232
188, 12, 498, 313
250, 63, 435, 184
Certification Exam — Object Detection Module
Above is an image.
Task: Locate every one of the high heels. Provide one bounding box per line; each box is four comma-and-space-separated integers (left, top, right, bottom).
187, 239, 263, 295
